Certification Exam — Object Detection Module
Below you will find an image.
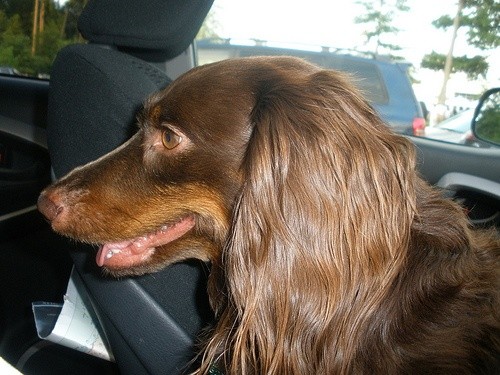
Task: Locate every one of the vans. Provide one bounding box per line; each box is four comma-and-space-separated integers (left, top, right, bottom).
193, 38, 426, 137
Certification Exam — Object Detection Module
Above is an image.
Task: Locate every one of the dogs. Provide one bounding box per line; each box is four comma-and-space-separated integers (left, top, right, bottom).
37, 56, 500, 375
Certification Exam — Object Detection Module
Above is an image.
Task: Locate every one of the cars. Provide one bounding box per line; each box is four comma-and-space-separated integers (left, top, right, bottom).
424, 104, 500, 150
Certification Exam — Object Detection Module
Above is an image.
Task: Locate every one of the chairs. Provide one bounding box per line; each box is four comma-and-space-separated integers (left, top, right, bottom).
46, 0, 215, 375
